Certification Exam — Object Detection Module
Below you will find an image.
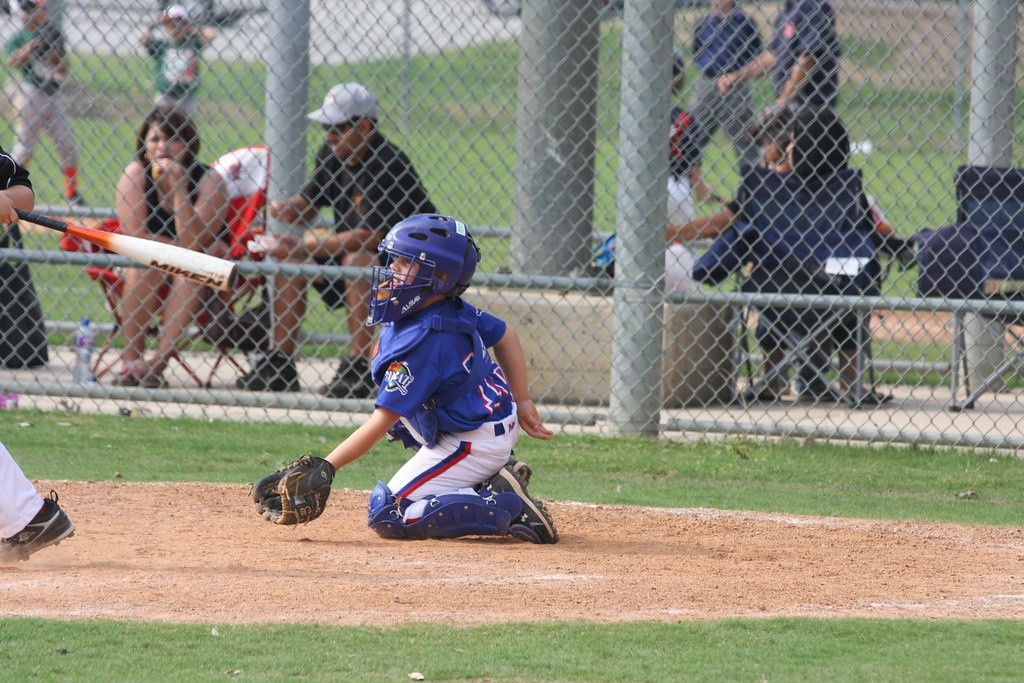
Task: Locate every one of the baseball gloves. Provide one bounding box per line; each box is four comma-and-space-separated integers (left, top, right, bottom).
246, 452, 336, 531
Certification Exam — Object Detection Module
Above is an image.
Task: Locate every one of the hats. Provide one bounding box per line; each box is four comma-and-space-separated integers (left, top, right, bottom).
162, 6, 190, 25
8, 0, 47, 18
307, 83, 378, 124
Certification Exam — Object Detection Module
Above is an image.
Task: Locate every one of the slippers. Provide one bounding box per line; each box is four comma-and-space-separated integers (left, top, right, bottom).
740, 380, 779, 402
838, 387, 893, 407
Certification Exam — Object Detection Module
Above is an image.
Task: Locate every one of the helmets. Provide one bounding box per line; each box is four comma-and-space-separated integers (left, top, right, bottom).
365, 214, 477, 326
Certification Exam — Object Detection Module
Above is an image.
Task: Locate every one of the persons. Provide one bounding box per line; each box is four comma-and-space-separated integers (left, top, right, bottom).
138, 4, 219, 125
251, 214, 560, 544
112, 104, 229, 389
0, 0, 92, 208
236, 81, 439, 399
0, 145, 74, 564
593, 0, 896, 404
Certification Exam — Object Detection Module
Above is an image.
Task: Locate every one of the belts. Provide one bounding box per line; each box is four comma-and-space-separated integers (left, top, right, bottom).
493, 422, 505, 437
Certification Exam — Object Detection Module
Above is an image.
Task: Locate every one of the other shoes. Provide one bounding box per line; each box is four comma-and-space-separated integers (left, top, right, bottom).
796, 379, 842, 403
68, 191, 89, 207
111, 364, 139, 387
126, 350, 164, 386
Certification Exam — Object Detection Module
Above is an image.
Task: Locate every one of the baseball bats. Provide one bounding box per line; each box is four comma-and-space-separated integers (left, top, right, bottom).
14, 206, 240, 293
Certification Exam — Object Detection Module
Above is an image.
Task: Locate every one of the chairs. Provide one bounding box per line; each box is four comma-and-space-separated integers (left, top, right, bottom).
692, 162, 904, 408
911, 162, 1023, 410
59, 145, 272, 389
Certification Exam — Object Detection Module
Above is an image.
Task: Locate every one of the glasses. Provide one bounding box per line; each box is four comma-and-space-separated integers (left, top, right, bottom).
322, 123, 345, 134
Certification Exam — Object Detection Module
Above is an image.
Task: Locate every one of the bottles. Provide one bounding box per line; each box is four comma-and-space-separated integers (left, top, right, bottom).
74, 317, 93, 385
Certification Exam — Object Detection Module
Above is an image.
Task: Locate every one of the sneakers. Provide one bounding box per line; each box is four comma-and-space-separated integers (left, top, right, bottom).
237, 348, 300, 392
487, 463, 557, 544
318, 356, 375, 400
478, 457, 532, 491
0, 490, 75, 563
